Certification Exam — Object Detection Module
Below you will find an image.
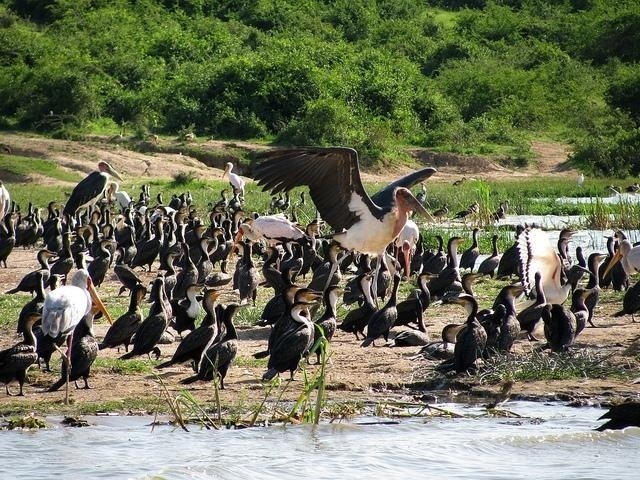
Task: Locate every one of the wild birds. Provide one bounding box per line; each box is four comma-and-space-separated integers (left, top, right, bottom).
592, 401, 639, 433
0, 144, 640, 398
